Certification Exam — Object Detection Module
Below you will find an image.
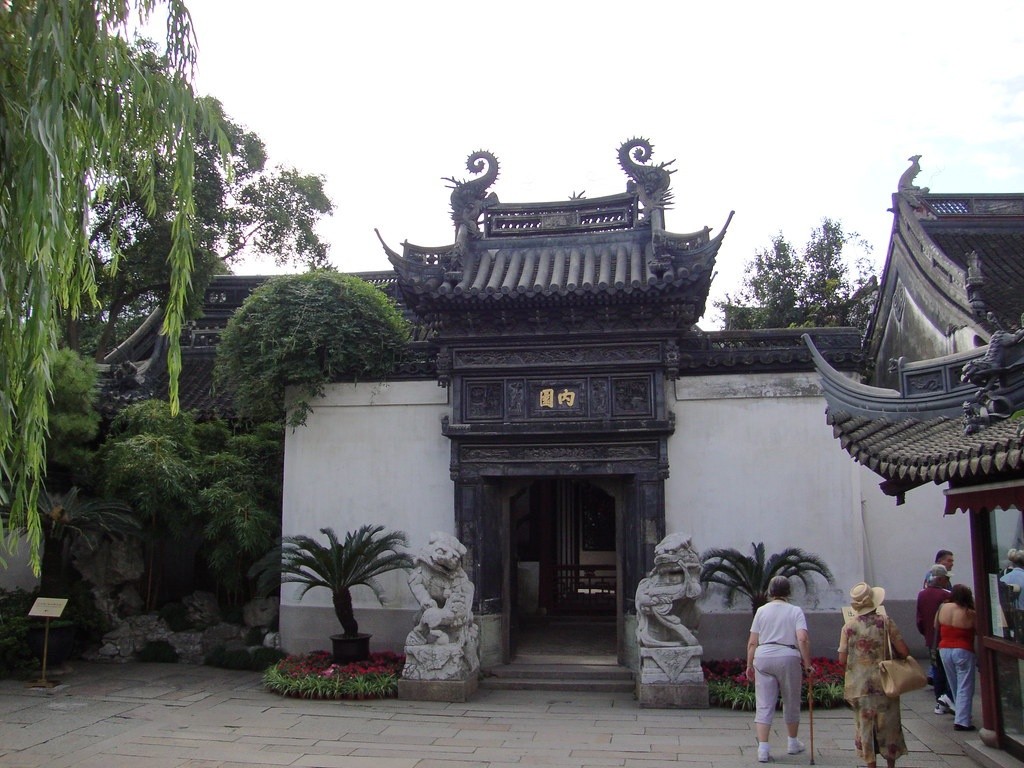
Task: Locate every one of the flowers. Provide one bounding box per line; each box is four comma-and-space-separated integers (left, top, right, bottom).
278, 650, 405, 677
701, 658, 845, 685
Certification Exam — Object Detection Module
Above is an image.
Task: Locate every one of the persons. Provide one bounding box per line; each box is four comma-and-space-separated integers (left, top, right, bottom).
924, 549, 954, 686
915, 563, 957, 716
839, 582, 910, 768
932, 584, 981, 732
745, 575, 816, 762
998, 548, 1024, 613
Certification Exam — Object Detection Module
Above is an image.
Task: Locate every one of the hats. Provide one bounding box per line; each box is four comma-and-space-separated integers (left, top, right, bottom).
850, 582, 885, 617
932, 565, 954, 578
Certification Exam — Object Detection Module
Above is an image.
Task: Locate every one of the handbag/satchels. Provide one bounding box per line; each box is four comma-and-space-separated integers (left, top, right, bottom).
878, 654, 929, 697
931, 647, 943, 672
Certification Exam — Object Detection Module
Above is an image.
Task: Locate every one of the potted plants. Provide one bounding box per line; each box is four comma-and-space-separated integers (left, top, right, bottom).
709, 680, 845, 711
258, 524, 415, 664
25, 591, 75, 667
262, 659, 397, 700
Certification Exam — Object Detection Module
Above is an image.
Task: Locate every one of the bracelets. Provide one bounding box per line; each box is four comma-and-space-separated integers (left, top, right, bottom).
746, 665, 753, 667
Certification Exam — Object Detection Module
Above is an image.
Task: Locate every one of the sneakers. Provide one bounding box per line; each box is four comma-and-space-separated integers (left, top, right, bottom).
938, 694, 955, 715
934, 699, 949, 714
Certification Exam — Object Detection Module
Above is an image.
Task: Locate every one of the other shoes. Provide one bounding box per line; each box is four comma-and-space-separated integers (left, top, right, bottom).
757, 749, 768, 762
787, 741, 805, 754
953, 724, 976, 731
